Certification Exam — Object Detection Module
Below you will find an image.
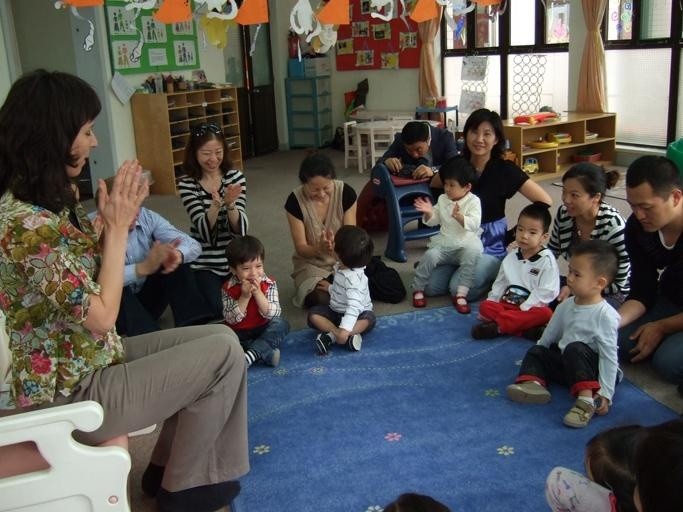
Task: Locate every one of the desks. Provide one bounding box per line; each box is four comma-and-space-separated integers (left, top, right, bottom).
412, 99, 461, 162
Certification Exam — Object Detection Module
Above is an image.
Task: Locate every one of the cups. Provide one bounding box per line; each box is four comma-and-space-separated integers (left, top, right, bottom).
153, 78, 163, 95
165, 80, 173, 94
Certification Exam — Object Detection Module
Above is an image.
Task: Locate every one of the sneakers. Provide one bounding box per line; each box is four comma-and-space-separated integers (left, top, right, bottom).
563, 400, 597, 428
505, 381, 551, 404
345, 334, 362, 351
524, 326, 545, 340
315, 333, 332, 355
472, 320, 497, 339
263, 348, 280, 366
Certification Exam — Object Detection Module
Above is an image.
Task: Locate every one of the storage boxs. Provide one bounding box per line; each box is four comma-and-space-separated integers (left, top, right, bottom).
284, 33, 332, 80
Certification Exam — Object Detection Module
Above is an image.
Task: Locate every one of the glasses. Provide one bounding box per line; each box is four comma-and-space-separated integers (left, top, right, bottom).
190, 123, 221, 137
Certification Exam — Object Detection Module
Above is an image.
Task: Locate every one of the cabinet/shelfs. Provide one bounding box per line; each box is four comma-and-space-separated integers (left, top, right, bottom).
283, 75, 335, 150
495, 106, 623, 188
126, 83, 245, 197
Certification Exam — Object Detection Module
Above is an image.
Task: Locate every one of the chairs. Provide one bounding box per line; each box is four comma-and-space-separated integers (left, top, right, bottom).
334, 109, 458, 178
1, 303, 164, 512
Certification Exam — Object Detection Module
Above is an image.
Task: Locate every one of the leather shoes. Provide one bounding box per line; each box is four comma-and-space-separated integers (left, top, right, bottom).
413, 290, 426, 307
453, 294, 471, 314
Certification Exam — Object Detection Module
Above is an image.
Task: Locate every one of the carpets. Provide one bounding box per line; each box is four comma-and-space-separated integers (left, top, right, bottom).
223, 295, 682, 511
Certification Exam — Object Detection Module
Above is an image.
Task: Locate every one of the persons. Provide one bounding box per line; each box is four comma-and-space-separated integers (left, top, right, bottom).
178, 122, 291, 367
381, 108, 683, 426
284, 151, 405, 354
83, 176, 214, 339
545, 414, 683, 512
1, 69, 251, 511
